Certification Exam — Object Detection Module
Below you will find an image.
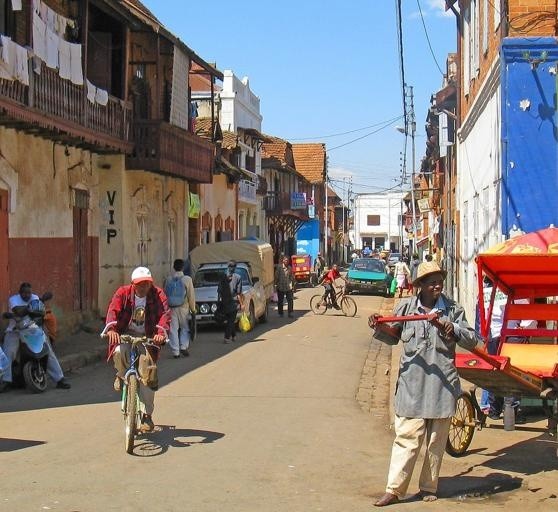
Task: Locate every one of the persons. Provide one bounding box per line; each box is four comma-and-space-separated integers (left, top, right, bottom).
313, 253, 325, 284
216, 260, 245, 343
369, 261, 477, 506
322, 262, 350, 310
483, 272, 527, 423
475, 272, 490, 415
164, 259, 195, 360
106, 266, 172, 435
371, 248, 441, 298
274, 255, 296, 317
3, 284, 73, 391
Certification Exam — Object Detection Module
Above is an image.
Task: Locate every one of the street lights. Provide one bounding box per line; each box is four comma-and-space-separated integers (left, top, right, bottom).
391, 176, 414, 188
394, 125, 417, 252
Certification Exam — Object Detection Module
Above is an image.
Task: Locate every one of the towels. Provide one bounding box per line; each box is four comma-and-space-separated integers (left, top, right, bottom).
1, 0, 109, 106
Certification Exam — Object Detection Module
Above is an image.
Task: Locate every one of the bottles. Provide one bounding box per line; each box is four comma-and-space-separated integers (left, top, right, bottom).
502, 397, 520, 430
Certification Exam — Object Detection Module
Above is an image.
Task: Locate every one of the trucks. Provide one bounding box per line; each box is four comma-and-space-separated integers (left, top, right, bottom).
184, 236, 276, 329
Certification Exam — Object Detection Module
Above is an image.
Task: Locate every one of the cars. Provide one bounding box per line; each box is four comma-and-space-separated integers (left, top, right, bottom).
343, 258, 389, 296
387, 253, 402, 268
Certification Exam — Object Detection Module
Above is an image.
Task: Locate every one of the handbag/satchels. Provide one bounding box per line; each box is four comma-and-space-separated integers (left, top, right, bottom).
138, 353, 159, 388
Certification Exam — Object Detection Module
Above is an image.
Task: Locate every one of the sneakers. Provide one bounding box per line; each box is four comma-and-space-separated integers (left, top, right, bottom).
224, 335, 238, 344
113, 376, 123, 392
56, 381, 71, 389
140, 413, 154, 432
181, 349, 189, 358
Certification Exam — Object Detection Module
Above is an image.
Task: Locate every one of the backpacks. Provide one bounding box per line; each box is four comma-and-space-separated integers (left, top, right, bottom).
317, 269, 331, 284
165, 275, 189, 307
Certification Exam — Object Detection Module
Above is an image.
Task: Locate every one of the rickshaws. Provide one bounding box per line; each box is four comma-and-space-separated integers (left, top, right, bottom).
370, 226, 557, 455
290, 253, 314, 288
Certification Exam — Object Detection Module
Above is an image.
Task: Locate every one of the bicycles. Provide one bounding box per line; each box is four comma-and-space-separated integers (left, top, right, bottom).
97, 320, 170, 452
309, 282, 358, 318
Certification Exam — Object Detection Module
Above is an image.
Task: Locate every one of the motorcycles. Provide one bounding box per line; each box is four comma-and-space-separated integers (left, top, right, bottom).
1, 290, 54, 394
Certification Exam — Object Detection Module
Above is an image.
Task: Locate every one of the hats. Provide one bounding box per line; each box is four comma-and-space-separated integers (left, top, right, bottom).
131, 266, 153, 285
410, 261, 448, 287
